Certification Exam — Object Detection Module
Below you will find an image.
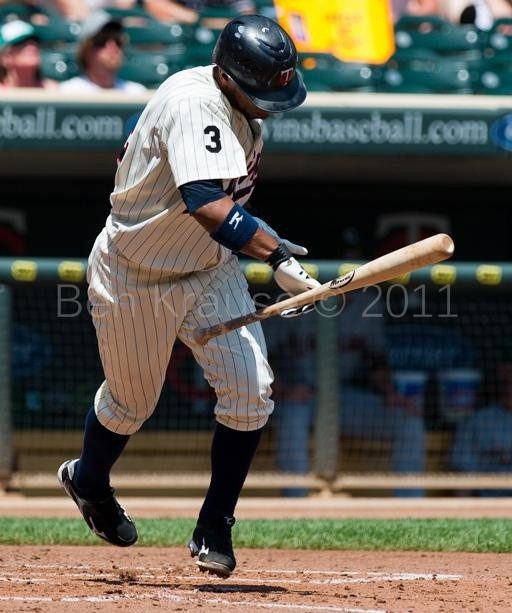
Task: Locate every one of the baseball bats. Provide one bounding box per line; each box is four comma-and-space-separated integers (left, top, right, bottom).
192, 233, 454, 347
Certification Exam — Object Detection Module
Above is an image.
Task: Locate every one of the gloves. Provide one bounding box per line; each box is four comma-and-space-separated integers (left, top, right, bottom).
266, 243, 325, 317
253, 217, 308, 255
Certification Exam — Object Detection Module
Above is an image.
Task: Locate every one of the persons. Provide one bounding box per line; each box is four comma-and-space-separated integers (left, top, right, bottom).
1, 18, 60, 88
57, 14, 324, 579
449, 360, 511, 496
272, 382, 314, 404
58, 9, 147, 90
2, 1, 511, 47
363, 366, 421, 417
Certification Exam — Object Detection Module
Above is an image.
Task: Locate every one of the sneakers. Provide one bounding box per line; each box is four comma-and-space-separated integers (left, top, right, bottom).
58, 458, 137, 547
188, 513, 237, 578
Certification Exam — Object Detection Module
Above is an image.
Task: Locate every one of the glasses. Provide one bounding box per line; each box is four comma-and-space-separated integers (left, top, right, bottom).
92, 34, 123, 50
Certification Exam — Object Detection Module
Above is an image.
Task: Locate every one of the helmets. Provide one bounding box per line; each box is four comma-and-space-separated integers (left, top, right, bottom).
212, 13, 307, 112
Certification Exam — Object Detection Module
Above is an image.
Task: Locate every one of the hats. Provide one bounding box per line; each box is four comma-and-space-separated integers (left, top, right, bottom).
0, 19, 38, 50
77, 10, 125, 65
